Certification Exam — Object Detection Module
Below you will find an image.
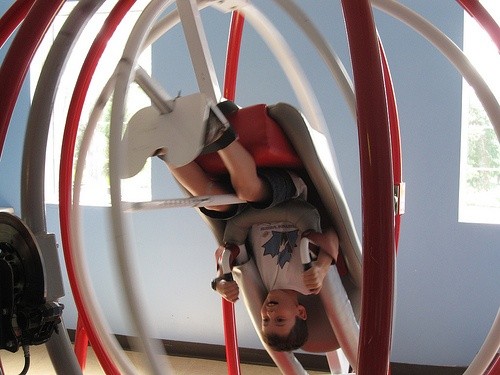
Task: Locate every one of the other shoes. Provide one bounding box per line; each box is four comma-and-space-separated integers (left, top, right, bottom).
204, 107, 223, 147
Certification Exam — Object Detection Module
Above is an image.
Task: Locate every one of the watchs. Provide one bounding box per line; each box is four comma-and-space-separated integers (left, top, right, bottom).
211, 277, 223, 290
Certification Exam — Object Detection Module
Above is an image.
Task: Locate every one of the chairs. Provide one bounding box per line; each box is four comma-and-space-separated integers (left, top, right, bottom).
174, 102, 366, 354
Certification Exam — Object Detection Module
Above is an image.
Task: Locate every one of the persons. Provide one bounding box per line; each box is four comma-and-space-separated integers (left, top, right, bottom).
151, 103, 332, 353
